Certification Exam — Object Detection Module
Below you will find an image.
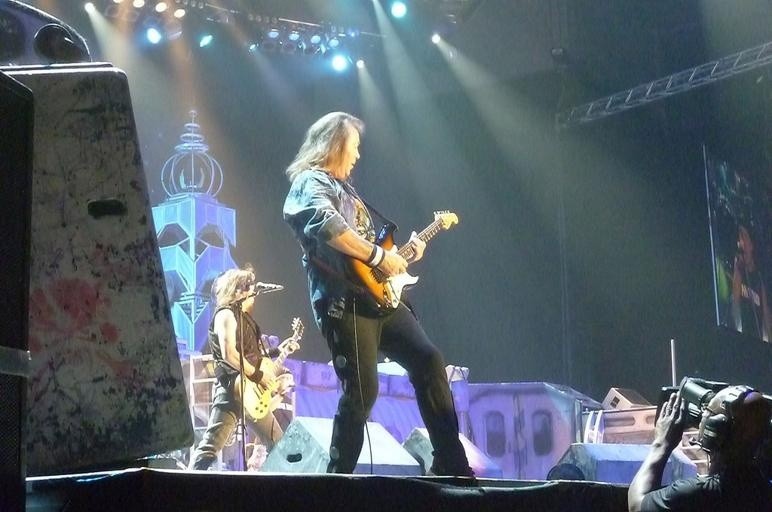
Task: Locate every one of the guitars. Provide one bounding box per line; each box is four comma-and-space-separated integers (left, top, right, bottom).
343, 210, 459, 314
234, 317, 305, 422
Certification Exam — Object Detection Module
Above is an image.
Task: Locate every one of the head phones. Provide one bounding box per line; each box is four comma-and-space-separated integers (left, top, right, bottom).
699, 384, 759, 450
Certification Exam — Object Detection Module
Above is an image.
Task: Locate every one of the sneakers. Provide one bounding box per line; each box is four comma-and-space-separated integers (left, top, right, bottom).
426, 449, 477, 477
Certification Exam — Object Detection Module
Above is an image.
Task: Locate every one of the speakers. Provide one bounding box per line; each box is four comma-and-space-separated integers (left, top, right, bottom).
0, 65, 36, 512
547, 442, 673, 485
259, 416, 421, 476
401, 427, 504, 478
12, 68, 199, 480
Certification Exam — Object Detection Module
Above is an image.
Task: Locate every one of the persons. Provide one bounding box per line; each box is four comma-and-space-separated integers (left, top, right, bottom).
187, 268, 301, 471
282, 109, 474, 476
627, 386, 772, 511
732, 221, 771, 343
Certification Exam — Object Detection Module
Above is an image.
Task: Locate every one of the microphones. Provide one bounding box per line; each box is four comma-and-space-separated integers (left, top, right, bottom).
255, 282, 285, 291
689, 437, 712, 453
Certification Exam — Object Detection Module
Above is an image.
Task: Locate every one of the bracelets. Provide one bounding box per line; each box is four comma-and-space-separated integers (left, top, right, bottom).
366, 243, 385, 268
250, 369, 263, 383
269, 346, 279, 357
